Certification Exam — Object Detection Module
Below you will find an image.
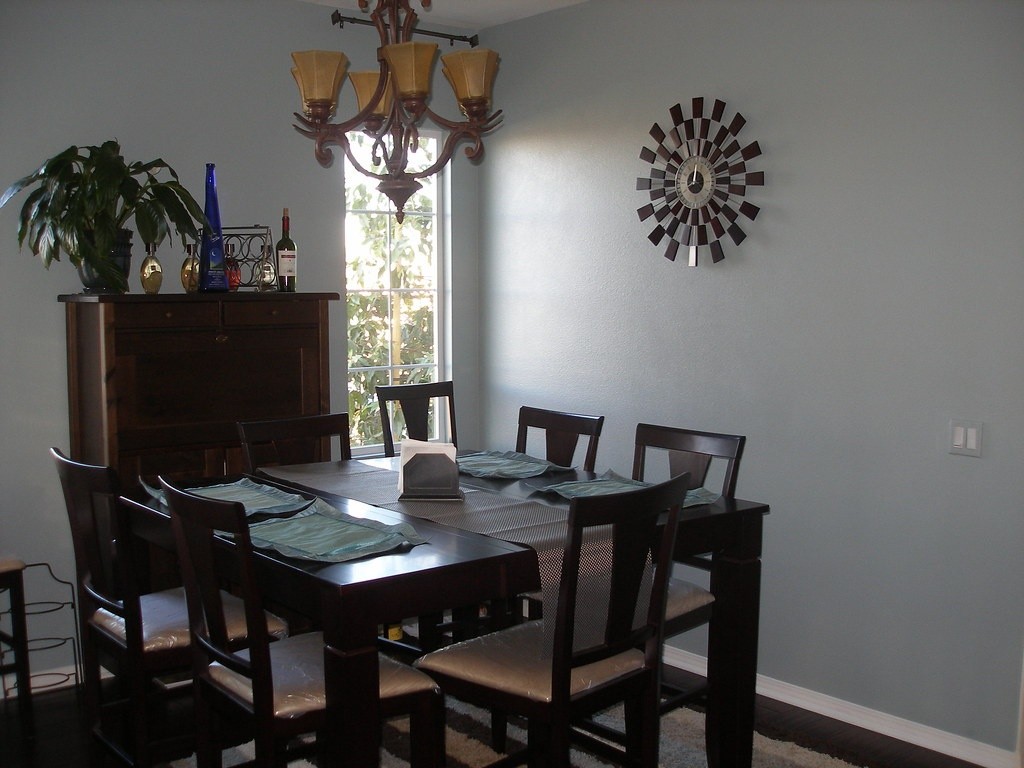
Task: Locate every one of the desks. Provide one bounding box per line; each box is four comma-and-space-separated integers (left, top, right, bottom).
121, 449, 771, 768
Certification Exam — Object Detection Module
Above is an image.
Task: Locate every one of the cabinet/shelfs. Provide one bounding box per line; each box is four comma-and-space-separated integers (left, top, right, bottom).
56, 292, 338, 599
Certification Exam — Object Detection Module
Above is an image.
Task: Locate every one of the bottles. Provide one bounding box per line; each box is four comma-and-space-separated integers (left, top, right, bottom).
200, 163, 226, 293
223, 244, 241, 293
181, 244, 200, 293
140, 243, 163, 293
256, 245, 278, 292
276, 208, 297, 292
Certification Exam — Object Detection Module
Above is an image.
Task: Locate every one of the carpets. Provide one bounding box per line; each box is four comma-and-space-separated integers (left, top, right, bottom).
160, 693, 869, 768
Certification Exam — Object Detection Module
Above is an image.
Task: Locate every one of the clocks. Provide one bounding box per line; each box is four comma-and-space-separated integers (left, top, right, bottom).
634, 95, 766, 269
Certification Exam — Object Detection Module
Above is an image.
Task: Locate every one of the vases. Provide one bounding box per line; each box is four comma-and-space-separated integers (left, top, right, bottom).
197, 162, 232, 293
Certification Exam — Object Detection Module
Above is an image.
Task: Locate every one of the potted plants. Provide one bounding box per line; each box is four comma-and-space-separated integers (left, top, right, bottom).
0, 136, 214, 295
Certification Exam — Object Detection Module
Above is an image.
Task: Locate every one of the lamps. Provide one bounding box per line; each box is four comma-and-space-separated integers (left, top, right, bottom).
289, 0, 503, 224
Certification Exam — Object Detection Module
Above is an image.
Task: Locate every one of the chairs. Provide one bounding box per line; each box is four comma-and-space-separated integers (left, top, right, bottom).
48, 447, 290, 768
233, 413, 353, 473
489, 423, 748, 748
374, 379, 459, 457
401, 405, 606, 643
409, 469, 694, 768
158, 473, 447, 768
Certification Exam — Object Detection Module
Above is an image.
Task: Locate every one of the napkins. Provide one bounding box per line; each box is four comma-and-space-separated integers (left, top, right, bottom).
212, 498, 433, 564
138, 474, 317, 517
524, 466, 723, 509
456, 449, 579, 479
